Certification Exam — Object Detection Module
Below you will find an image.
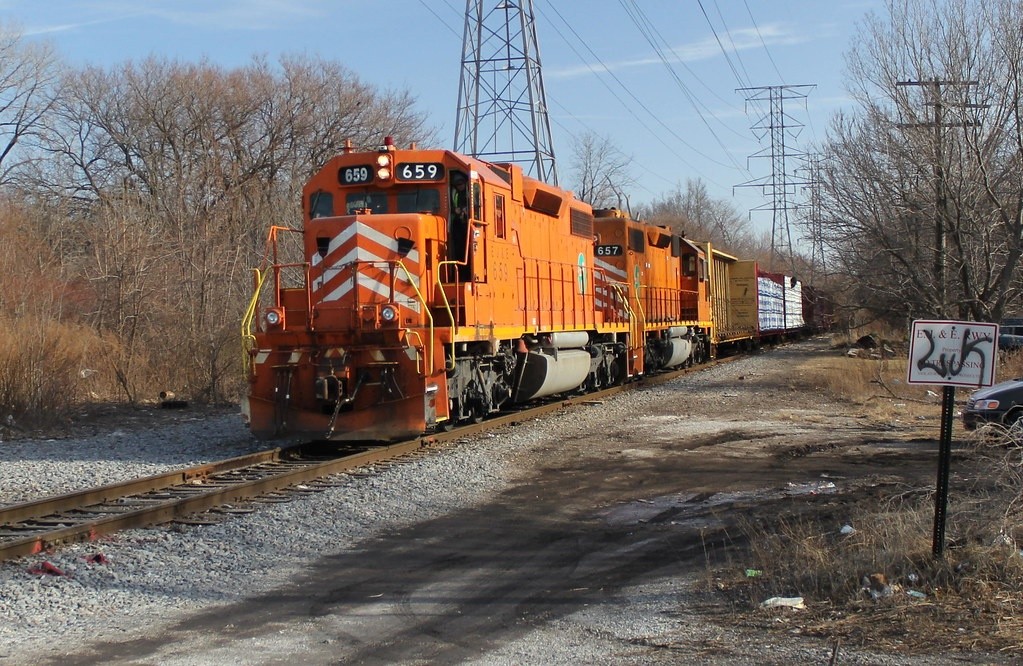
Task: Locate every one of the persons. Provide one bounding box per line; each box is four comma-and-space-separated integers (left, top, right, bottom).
451, 175, 468, 262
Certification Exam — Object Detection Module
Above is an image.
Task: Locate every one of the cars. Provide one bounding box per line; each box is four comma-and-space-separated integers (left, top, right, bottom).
959, 377, 1023, 447
998, 325, 1023, 353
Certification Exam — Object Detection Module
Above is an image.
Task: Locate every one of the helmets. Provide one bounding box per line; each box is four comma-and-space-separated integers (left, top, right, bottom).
451, 173, 466, 185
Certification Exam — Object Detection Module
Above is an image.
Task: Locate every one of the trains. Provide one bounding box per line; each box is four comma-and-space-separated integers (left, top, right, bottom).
239, 132, 838, 444
